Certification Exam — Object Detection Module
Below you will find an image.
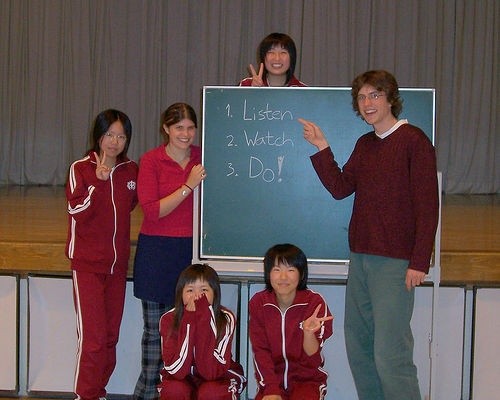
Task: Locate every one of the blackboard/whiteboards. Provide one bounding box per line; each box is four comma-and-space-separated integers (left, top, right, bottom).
200, 86, 435, 262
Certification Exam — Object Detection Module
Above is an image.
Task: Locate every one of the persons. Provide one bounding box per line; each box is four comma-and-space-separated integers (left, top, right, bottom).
248, 242, 336, 400
132, 103, 206, 400
64, 108, 138, 400
239, 32, 304, 88
297, 70, 440, 400
159, 262, 248, 400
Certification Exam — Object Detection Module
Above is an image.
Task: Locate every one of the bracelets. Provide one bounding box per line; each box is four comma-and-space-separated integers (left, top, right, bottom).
183, 183, 194, 192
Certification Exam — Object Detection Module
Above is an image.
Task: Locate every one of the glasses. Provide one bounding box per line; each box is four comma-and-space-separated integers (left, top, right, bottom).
356, 93, 388, 101
103, 133, 129, 144
